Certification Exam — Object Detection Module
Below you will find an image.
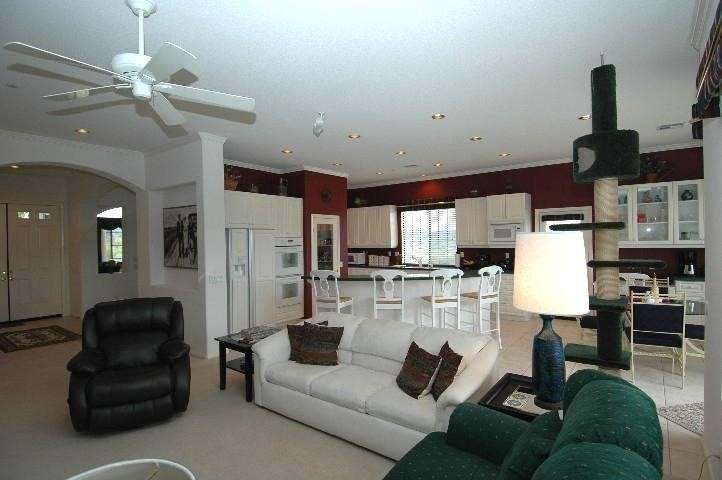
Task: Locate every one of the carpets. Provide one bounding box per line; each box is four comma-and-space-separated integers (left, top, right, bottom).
0, 325, 81, 353
657, 401, 704, 437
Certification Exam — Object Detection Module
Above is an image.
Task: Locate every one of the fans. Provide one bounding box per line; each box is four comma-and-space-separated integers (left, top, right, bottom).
3, 0, 254, 126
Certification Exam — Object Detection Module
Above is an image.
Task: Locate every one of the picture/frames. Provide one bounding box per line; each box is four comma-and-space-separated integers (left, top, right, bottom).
162, 205, 198, 269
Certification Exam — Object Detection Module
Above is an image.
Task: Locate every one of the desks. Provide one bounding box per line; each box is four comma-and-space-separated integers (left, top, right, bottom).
478, 372, 563, 423
214, 326, 283, 402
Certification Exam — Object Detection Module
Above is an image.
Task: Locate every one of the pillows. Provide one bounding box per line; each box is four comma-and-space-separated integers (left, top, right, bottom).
495, 410, 562, 480
286, 320, 465, 403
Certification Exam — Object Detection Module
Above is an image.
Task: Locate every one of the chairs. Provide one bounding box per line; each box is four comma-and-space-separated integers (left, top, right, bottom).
308, 264, 503, 353
67, 297, 191, 434
575, 273, 705, 389
383, 370, 664, 480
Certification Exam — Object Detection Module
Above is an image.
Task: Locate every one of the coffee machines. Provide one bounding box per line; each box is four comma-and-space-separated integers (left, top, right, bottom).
680, 249, 697, 278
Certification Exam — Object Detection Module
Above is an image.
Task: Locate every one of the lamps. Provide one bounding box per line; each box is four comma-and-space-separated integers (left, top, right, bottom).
513, 231, 590, 410
313, 112, 326, 136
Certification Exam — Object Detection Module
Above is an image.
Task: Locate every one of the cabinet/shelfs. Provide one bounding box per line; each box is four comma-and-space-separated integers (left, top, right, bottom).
224, 190, 305, 325
455, 193, 533, 249
347, 205, 398, 248
617, 180, 704, 248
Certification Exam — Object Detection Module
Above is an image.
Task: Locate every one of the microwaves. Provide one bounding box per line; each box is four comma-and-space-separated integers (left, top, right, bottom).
489, 223, 521, 242
348, 253, 367, 265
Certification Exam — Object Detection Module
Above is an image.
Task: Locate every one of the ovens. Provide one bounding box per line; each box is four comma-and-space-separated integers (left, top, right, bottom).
275, 276, 304, 308
274, 238, 305, 276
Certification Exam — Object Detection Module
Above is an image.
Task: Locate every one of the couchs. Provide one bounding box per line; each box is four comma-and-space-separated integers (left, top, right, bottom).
251, 311, 503, 463
